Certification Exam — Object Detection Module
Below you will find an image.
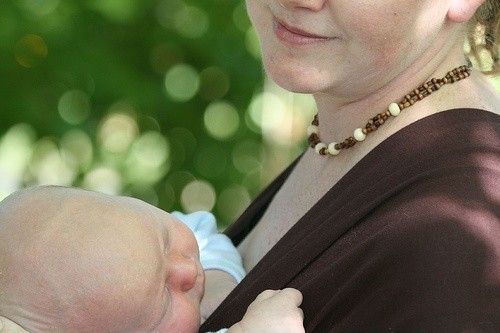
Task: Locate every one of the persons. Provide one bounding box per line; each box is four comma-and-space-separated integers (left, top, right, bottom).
0, 183, 305, 333
199, 0, 500, 333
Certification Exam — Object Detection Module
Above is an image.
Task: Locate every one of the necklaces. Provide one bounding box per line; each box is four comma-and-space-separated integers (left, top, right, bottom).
306, 64, 474, 156
